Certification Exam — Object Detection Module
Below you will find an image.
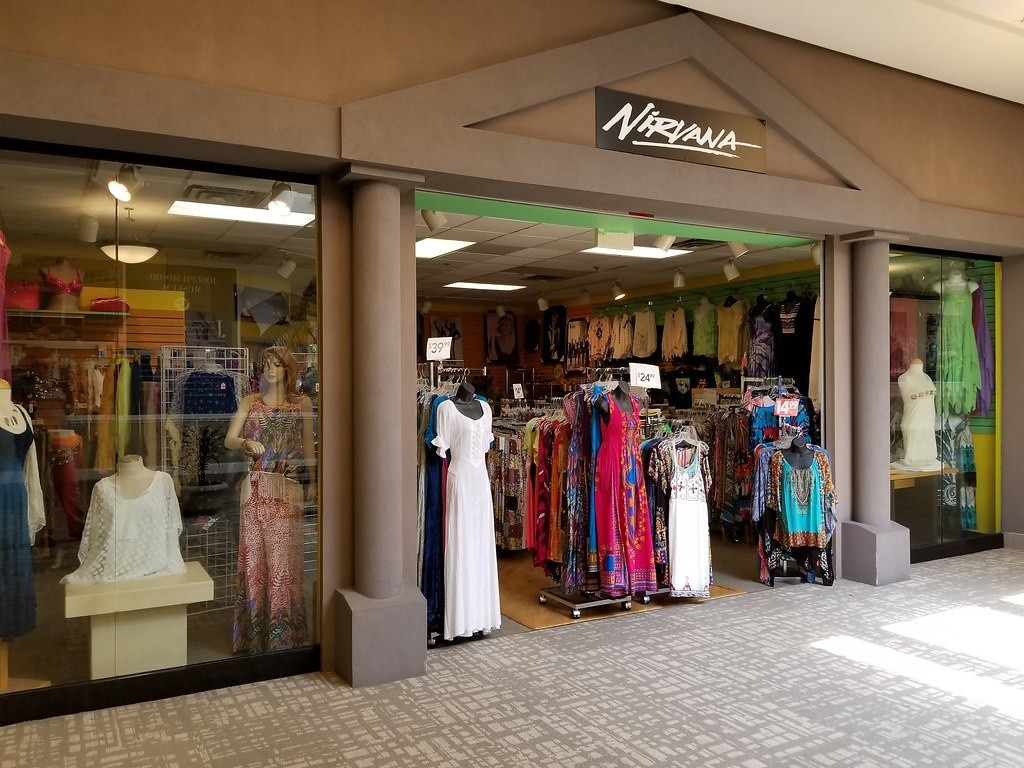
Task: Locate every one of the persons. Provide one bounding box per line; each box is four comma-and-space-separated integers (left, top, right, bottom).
898, 358, 938, 468
39, 256, 85, 311
936, 410, 970, 511
594, 379, 659, 598
222, 345, 317, 650
765, 434, 838, 548
548, 311, 562, 359
928, 270, 982, 415
8, 321, 86, 571
489, 315, 516, 362
0, 379, 47, 640
432, 380, 503, 642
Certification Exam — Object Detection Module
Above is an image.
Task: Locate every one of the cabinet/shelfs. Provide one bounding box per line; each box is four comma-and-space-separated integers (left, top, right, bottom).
890, 464, 959, 549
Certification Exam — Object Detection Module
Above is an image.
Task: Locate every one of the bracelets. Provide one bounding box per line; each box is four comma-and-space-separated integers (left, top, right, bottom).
241, 438, 254, 454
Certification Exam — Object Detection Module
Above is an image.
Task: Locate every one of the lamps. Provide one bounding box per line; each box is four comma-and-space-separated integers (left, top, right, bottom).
268, 180, 293, 214
421, 296, 433, 315
811, 243, 820, 266
277, 252, 296, 279
75, 213, 99, 243
95, 218, 161, 264
421, 210, 448, 232
108, 164, 146, 203
495, 235, 750, 317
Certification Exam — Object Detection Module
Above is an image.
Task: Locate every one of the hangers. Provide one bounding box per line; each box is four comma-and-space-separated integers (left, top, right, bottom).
742, 376, 802, 401
47, 347, 151, 371
774, 422, 803, 449
416, 363, 477, 406
563, 367, 629, 406
199, 347, 222, 372
655, 394, 740, 439
493, 397, 564, 420
